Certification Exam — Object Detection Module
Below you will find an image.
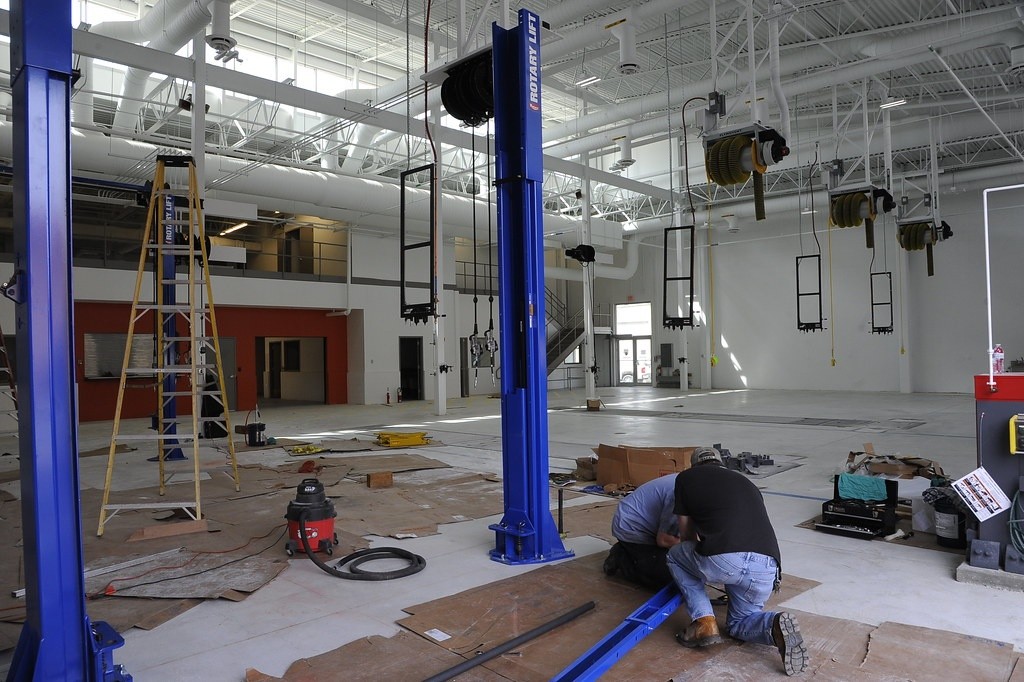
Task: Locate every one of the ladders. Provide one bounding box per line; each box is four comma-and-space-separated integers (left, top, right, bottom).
0, 322, 21, 485
97, 154, 241, 537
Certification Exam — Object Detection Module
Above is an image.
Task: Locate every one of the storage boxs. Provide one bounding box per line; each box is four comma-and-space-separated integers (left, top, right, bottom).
590, 443, 702, 490
860, 442, 917, 479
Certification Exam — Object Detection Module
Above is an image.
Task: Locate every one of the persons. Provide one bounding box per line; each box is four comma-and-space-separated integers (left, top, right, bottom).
665, 447, 809, 678
602, 473, 683, 587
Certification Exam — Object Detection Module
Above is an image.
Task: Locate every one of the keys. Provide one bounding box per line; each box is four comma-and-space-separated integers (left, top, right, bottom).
773, 580, 781, 595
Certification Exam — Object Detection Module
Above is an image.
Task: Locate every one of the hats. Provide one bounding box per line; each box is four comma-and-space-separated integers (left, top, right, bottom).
691, 446, 722, 466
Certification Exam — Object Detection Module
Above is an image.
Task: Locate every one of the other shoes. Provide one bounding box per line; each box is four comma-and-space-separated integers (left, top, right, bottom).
604, 541, 623, 576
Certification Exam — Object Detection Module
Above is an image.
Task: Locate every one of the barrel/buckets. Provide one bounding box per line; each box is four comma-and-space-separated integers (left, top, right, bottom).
934, 497, 976, 548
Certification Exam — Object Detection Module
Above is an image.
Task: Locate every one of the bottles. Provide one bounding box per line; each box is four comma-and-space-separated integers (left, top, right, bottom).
992, 344, 1004, 374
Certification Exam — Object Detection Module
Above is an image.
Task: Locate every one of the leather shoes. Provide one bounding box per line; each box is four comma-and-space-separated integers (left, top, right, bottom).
676, 618, 723, 648
772, 612, 809, 677
